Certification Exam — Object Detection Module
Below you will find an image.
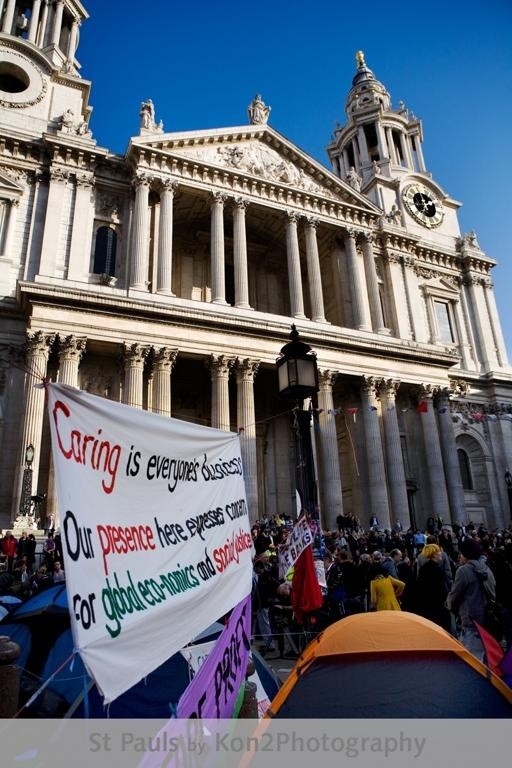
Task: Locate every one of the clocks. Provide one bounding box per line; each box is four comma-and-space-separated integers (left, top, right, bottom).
401, 182, 446, 229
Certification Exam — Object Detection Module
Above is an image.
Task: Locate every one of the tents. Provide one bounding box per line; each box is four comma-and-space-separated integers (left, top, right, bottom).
0, 585, 512, 768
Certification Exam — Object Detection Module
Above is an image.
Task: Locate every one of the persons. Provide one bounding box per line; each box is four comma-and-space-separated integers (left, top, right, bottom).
59, 108, 76, 135
248, 94, 272, 125
468, 228, 481, 249
138, 99, 155, 128
0, 511, 512, 767
385, 204, 403, 226
347, 165, 362, 193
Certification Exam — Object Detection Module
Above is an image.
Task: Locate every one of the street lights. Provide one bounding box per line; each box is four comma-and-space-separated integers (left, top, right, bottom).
275, 323, 318, 523
504, 467, 511, 517
405, 478, 419, 530
19, 444, 34, 514
